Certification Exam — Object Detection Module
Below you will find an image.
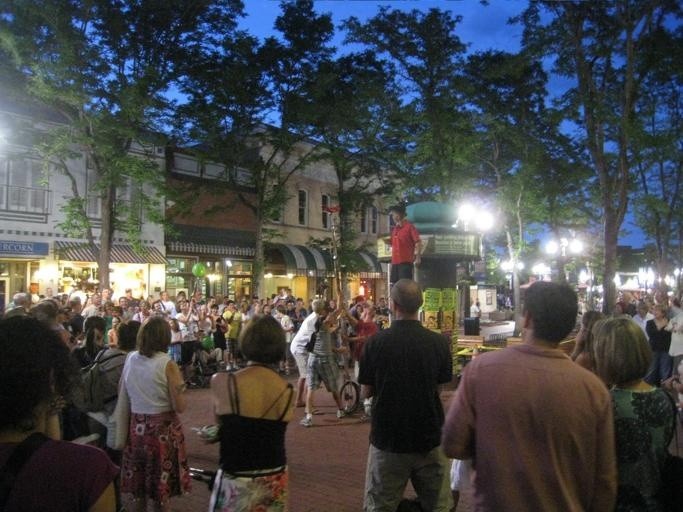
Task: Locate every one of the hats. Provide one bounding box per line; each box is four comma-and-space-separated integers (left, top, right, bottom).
388, 205, 408, 217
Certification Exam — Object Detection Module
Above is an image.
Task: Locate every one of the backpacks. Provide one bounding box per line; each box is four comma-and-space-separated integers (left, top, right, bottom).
70, 347, 126, 415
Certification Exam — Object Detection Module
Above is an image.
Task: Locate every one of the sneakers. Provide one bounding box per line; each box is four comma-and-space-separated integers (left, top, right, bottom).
337, 408, 348, 419
226, 365, 241, 371
298, 416, 313, 427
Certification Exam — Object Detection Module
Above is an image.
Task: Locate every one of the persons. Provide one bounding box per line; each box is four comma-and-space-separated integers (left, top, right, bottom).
377, 202, 422, 283
1, 276, 683, 512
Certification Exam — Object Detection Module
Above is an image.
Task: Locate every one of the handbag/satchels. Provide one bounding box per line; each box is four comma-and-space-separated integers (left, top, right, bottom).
105, 389, 130, 452
